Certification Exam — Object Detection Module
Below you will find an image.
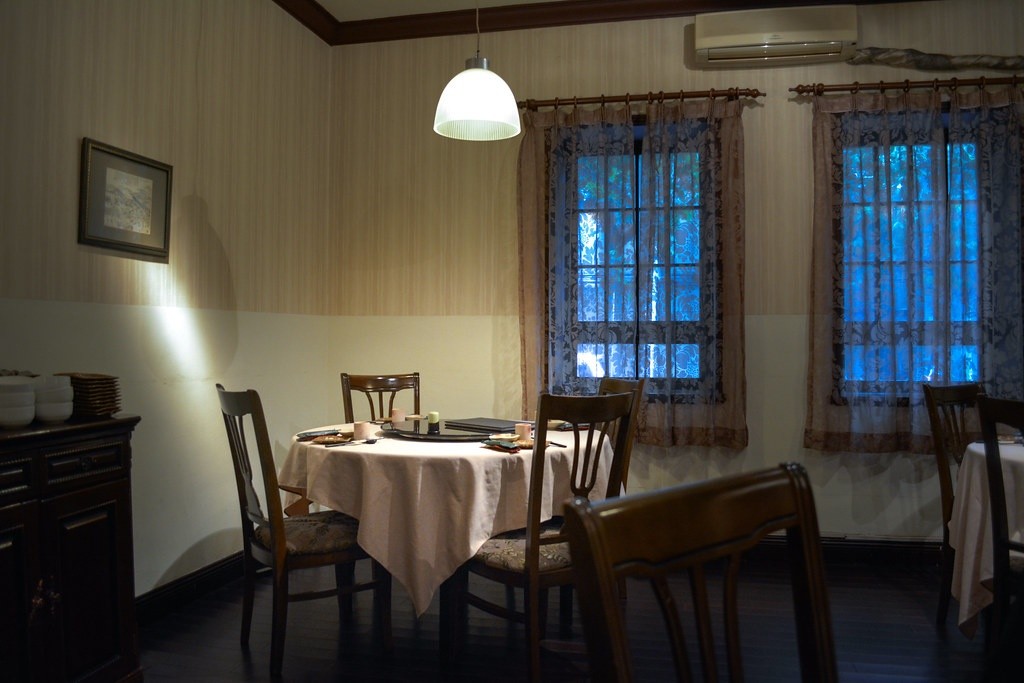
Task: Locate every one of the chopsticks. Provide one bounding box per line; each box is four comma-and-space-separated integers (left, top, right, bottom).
531, 437, 567, 448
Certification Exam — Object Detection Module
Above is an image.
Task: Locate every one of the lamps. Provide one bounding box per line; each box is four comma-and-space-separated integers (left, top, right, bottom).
432, 1, 524, 143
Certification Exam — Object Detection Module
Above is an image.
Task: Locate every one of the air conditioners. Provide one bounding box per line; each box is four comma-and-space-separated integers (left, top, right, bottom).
694, 5, 859, 70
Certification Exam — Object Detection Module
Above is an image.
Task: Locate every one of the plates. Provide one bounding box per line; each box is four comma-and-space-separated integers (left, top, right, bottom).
489, 434, 520, 441
53, 371, 123, 419
309, 436, 349, 443
514, 440, 550, 448
998, 440, 1014, 444
297, 429, 338, 438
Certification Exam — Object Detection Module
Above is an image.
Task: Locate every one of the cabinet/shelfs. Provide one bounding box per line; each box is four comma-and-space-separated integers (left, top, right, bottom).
0, 412, 148, 683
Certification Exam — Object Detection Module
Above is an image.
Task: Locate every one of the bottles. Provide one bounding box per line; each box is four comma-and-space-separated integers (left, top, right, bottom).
1014, 428, 1023, 443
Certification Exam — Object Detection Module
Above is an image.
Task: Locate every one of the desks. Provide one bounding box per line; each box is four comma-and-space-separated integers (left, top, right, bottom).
276, 417, 628, 682
945, 442, 1024, 641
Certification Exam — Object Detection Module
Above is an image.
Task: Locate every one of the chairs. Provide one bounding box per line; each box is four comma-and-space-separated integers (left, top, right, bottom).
447, 388, 641, 682
340, 371, 420, 423
559, 459, 838, 683
976, 392, 1024, 682
529, 374, 644, 629
923, 380, 982, 625
215, 382, 394, 679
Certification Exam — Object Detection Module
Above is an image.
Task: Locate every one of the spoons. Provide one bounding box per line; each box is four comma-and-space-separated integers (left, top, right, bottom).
364, 437, 378, 444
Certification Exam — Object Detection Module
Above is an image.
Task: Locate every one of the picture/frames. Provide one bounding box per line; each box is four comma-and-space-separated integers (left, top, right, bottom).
79, 137, 173, 259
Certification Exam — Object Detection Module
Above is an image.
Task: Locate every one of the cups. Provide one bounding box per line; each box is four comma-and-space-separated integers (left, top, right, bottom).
354, 423, 364, 439
515, 423, 532, 440
392, 408, 399, 418
427, 412, 440, 434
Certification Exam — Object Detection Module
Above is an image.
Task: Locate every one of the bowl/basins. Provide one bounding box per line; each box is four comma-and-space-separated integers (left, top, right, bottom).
32, 375, 74, 421
548, 420, 565, 430
0, 376, 36, 426
